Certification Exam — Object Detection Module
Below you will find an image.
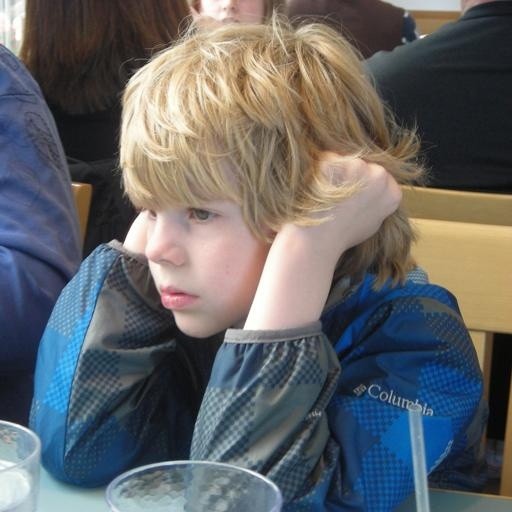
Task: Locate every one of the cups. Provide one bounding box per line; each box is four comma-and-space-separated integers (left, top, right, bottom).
102, 458, 284, 512
2, 418, 44, 511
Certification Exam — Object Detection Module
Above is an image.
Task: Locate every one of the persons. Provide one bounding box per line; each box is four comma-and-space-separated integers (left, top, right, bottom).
204, 1, 414, 58
357, 1, 510, 477
25, 8, 499, 510
3, 0, 203, 262
1, 46, 83, 424
